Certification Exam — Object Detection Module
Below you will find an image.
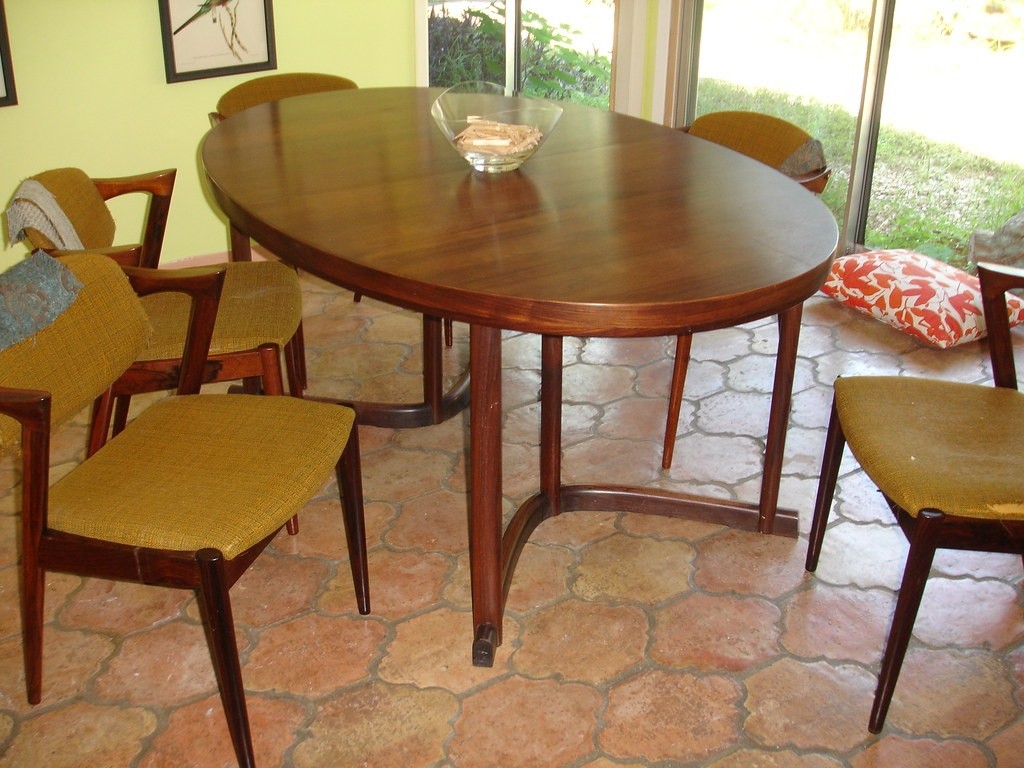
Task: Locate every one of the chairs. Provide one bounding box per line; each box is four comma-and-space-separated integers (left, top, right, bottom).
208, 72, 454, 347
0, 248, 371, 768
803, 260, 1024, 735
661, 111, 832, 472
3, 168, 309, 536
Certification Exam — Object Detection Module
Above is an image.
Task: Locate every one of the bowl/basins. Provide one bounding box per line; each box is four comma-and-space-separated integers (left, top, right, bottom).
433, 80, 563, 172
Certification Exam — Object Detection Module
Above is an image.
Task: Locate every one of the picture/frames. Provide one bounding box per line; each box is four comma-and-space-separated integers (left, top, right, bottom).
158, 0, 278, 84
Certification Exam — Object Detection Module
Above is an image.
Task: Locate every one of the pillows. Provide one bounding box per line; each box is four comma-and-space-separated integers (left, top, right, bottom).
820, 246, 1024, 349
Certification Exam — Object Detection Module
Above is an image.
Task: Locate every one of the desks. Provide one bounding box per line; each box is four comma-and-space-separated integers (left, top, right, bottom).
196, 87, 799, 667
